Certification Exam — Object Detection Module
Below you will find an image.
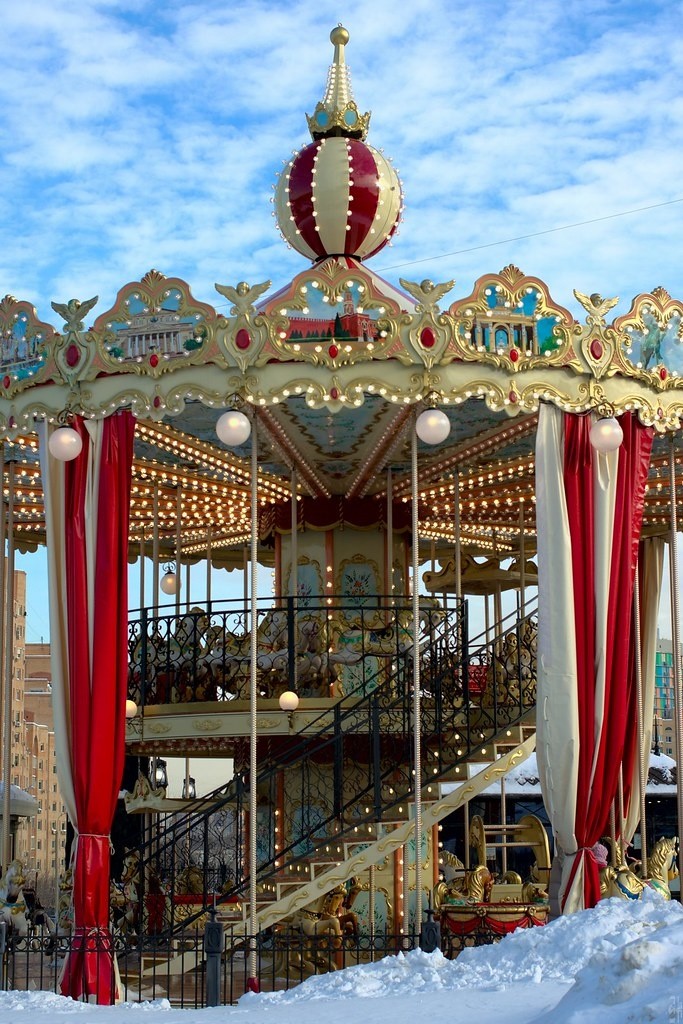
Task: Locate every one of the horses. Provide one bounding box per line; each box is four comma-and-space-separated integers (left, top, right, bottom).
120, 845, 359, 949
435, 850, 542, 910
127, 598, 445, 704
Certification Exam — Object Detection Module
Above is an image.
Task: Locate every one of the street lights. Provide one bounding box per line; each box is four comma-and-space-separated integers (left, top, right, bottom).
150, 757, 168, 876
182, 775, 196, 890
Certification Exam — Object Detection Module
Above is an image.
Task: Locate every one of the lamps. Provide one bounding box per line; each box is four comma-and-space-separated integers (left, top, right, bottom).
590, 401, 624, 453
160, 559, 182, 596
414, 389, 451, 444
49, 404, 82, 462
126, 699, 143, 734
278, 691, 300, 729
216, 391, 252, 447
183, 775, 196, 798
150, 758, 168, 789
